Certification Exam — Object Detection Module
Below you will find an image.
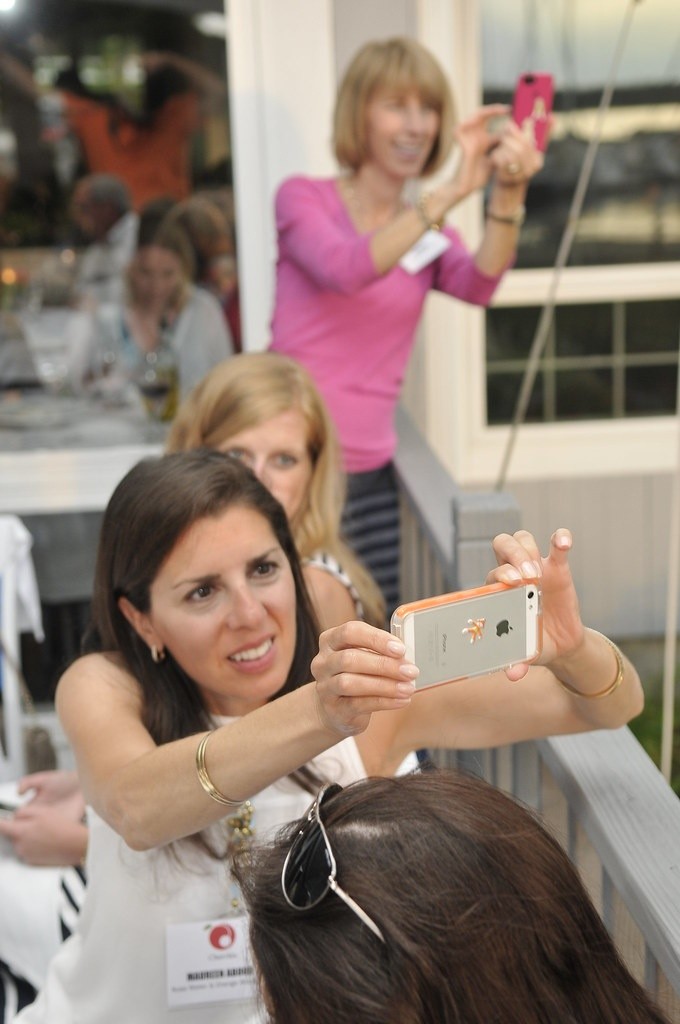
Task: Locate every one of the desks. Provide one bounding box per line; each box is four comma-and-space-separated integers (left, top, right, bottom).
0, 383, 180, 606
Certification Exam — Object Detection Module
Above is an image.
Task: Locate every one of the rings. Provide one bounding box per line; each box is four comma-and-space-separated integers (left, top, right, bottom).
506, 162, 521, 174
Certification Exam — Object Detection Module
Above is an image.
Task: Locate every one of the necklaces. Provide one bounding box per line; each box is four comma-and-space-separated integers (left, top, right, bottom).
227, 801, 264, 877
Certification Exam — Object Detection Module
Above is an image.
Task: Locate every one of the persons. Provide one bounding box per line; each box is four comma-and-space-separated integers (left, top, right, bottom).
11, 447, 646, 1024
243, 765, 671, 1024
0, 46, 226, 213
40, 171, 243, 430
0, 340, 392, 1024
268, 37, 549, 636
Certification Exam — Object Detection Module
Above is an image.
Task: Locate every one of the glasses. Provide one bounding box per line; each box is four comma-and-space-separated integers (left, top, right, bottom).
282, 783, 387, 947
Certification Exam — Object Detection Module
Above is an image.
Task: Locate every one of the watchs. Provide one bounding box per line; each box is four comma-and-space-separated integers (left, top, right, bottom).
483, 206, 527, 228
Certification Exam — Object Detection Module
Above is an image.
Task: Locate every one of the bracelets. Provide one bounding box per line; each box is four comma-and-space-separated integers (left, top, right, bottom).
157, 50, 176, 74
418, 188, 445, 230
195, 729, 247, 808
551, 626, 625, 700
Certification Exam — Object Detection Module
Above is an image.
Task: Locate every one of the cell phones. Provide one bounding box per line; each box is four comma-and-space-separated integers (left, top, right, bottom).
512, 73, 554, 152
390, 578, 543, 694
